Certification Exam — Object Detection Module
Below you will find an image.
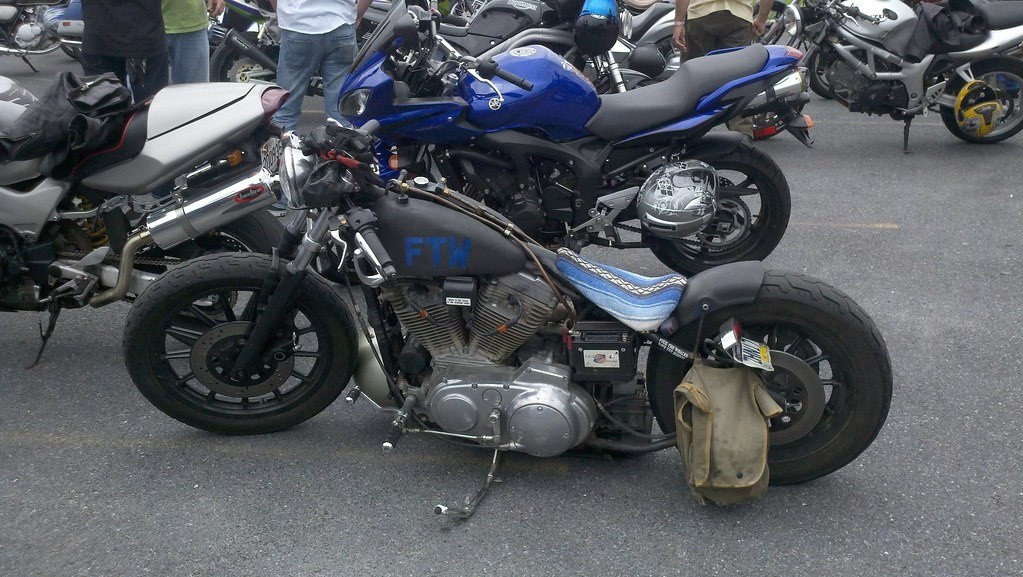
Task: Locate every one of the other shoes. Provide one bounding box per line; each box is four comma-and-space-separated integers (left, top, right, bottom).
266, 205, 285, 217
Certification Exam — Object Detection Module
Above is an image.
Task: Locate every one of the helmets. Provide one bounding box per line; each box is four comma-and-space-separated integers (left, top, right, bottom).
637, 159, 718, 239
574, 0, 620, 56
628, 43, 666, 79
953, 80, 1002, 137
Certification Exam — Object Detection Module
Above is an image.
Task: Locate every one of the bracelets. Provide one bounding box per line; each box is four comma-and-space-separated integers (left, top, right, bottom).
674, 21, 684, 25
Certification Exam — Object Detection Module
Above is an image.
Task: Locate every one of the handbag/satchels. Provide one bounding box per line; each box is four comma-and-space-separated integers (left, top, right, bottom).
674, 355, 783, 509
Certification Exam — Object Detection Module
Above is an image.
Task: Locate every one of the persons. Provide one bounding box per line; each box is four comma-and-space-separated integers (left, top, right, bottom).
670, 0, 774, 143
266, 0, 374, 216
79, 0, 225, 103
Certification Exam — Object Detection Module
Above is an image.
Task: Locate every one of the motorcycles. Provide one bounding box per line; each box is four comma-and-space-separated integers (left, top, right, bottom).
0, 0, 1023, 162
0, 70, 299, 373
119, 115, 897, 522
302, 0, 819, 278
